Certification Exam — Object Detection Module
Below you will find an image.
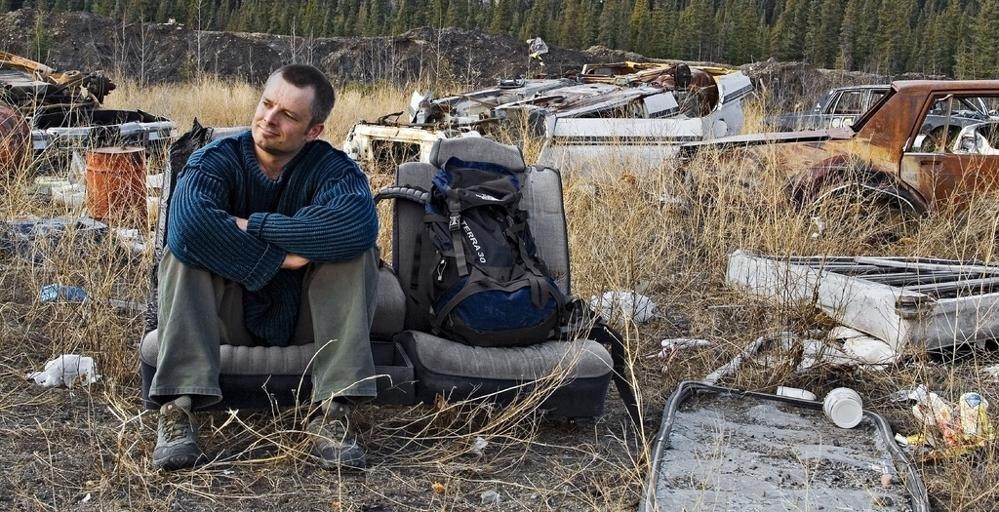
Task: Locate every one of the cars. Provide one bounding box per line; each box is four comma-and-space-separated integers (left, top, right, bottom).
2, 50, 173, 181
344, 68, 752, 168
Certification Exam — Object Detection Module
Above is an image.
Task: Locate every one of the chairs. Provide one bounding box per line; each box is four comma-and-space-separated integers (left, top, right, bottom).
138, 126, 406, 410
393, 137, 614, 419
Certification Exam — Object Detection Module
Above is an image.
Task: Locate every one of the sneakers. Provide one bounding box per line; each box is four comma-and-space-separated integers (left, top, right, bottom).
306, 416, 365, 470
152, 396, 206, 472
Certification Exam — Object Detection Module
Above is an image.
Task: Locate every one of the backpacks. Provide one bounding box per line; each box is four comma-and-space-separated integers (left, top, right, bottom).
376, 155, 567, 347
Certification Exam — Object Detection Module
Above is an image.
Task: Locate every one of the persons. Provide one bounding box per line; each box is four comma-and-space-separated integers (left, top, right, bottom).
147, 62, 379, 473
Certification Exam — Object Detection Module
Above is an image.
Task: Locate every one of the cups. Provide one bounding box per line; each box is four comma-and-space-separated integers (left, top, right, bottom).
821, 386, 864, 429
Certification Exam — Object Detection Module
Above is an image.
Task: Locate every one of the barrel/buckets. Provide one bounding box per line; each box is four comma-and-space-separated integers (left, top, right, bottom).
1, 101, 34, 179
86, 146, 148, 228
